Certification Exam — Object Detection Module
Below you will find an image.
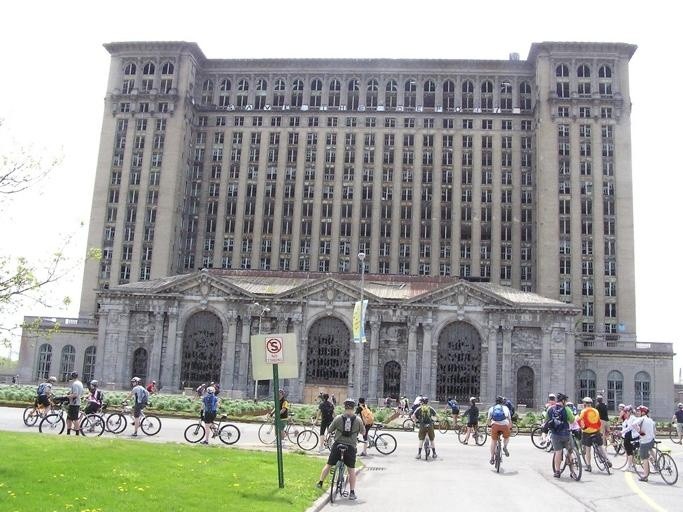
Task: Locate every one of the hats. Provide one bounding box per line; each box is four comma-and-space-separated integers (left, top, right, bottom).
677, 402, 682, 408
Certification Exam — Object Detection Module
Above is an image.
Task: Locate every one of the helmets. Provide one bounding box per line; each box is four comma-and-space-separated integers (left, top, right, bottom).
203, 382, 215, 393
420, 397, 428, 402
469, 396, 476, 401
91, 380, 97, 386
49, 377, 56, 382
70, 372, 77, 377
548, 393, 648, 413
343, 398, 354, 404
496, 395, 507, 404
131, 377, 141, 382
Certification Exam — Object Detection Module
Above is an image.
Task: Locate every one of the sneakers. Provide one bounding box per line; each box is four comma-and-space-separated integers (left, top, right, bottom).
200, 440, 208, 444
358, 452, 367, 456
490, 460, 495, 464
350, 494, 357, 499
502, 447, 510, 457
316, 481, 321, 487
415, 454, 421, 458
553, 457, 614, 479
433, 454, 437, 458
623, 465, 648, 481
132, 433, 137, 435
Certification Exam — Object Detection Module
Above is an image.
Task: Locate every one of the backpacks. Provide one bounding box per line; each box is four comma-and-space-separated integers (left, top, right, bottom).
452, 400, 459, 414
493, 406, 508, 421
37, 382, 51, 395
337, 415, 359, 438
91, 388, 104, 401
420, 406, 433, 424
138, 386, 149, 403
360, 404, 374, 426
545, 407, 565, 430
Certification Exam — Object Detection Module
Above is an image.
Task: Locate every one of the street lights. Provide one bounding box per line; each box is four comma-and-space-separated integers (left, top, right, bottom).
354, 251, 366, 406
253, 301, 271, 400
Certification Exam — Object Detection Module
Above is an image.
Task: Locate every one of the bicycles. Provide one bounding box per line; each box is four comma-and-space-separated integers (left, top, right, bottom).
184, 409, 240, 444
329, 443, 350, 503
402, 408, 519, 474
529, 398, 682, 485
23, 393, 161, 437
255, 410, 396, 454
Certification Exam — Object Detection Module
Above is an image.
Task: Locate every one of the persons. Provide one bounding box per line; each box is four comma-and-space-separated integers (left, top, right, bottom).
317, 398, 367, 500
542, 391, 656, 481
312, 389, 520, 464
200, 387, 218, 445
672, 402, 683, 445
12, 370, 156, 439
177, 381, 222, 395
268, 389, 290, 444
